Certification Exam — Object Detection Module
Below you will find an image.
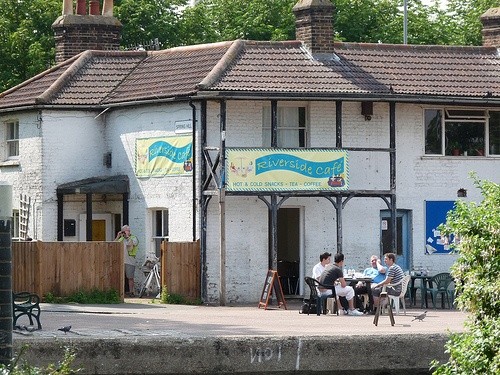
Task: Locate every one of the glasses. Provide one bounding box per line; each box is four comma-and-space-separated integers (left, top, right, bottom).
121, 231, 126, 234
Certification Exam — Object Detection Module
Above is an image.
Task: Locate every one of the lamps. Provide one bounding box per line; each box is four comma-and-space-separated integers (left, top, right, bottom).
360, 101, 373, 121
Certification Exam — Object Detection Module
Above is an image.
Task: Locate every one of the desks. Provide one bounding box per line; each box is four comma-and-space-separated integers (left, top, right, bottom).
344, 277, 374, 314
414, 274, 435, 309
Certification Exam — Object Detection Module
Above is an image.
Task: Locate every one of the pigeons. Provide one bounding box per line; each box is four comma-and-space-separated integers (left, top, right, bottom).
410, 310, 428, 322
58, 325, 72, 335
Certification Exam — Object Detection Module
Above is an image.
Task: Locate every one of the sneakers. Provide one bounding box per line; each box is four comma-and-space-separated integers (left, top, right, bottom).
348, 308, 364, 316
337, 308, 348, 315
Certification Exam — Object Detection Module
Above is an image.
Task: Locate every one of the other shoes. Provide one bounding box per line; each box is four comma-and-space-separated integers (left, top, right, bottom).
363, 309, 372, 316
130, 292, 135, 298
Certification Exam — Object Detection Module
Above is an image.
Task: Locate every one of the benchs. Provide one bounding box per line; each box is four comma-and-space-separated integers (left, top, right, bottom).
11, 290, 42, 331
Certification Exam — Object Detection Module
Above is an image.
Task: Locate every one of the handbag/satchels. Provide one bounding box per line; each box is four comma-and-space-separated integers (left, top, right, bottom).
302, 298, 321, 314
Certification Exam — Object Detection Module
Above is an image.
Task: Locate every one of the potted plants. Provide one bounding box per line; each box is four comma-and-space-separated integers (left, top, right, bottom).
471, 142, 484, 156
489, 133, 500, 154
450, 141, 462, 156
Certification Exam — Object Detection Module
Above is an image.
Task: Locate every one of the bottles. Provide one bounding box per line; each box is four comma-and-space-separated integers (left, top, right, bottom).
343, 267, 356, 279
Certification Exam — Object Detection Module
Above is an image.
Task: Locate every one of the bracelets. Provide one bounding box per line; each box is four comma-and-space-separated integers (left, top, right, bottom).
125, 237, 129, 239
117, 236, 119, 239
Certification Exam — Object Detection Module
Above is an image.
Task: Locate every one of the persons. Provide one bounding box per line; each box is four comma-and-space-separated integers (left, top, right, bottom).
319, 254, 364, 316
113, 225, 139, 298
313, 252, 335, 315
353, 255, 388, 312
369, 253, 405, 314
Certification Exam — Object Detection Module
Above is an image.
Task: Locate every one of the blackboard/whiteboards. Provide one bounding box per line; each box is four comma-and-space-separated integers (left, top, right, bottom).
260, 269, 287, 305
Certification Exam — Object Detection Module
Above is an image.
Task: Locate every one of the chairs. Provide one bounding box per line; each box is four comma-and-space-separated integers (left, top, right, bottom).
425, 272, 455, 310
382, 275, 411, 316
304, 277, 339, 317
